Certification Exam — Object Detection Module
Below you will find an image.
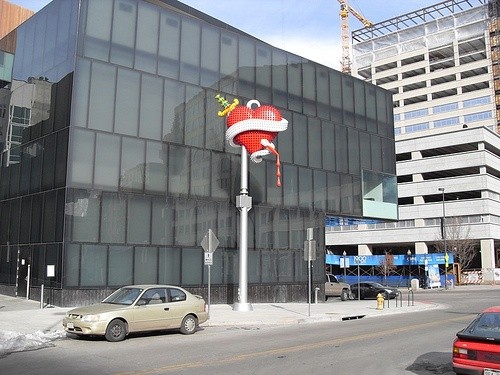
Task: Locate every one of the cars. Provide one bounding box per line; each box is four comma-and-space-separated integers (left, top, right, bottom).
62, 284, 208, 342
350, 282, 399, 300
452, 306, 500, 375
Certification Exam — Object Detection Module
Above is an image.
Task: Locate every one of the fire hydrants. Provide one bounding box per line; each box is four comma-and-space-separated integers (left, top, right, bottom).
376, 292, 384, 310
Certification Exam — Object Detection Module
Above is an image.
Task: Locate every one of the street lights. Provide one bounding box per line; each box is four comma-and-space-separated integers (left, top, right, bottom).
343, 251, 347, 283
407, 249, 411, 288
438, 188, 448, 290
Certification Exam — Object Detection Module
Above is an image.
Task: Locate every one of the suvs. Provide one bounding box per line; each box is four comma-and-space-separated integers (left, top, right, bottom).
325, 274, 352, 301
